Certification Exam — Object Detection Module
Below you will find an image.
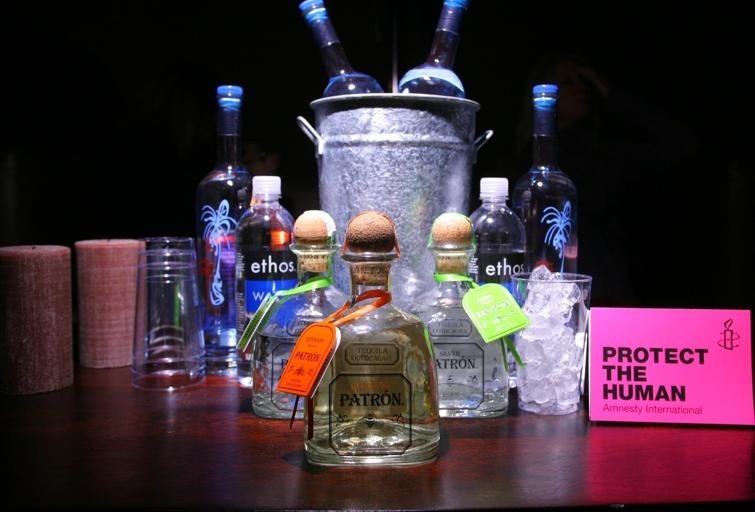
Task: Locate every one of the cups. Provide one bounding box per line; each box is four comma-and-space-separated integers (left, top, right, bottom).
511, 271, 592, 415
130, 237, 207, 390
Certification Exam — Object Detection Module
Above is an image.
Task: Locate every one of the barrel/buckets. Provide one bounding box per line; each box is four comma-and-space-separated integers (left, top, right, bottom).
297, 91, 494, 302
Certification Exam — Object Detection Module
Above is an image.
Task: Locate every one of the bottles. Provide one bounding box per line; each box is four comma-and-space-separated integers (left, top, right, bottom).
303, 210, 442, 467
299, 0, 384, 96
399, 0, 470, 97
237, 175, 298, 389
252, 210, 350, 420
197, 82, 251, 377
404, 212, 509, 418
512, 84, 580, 274
468, 177, 526, 391
